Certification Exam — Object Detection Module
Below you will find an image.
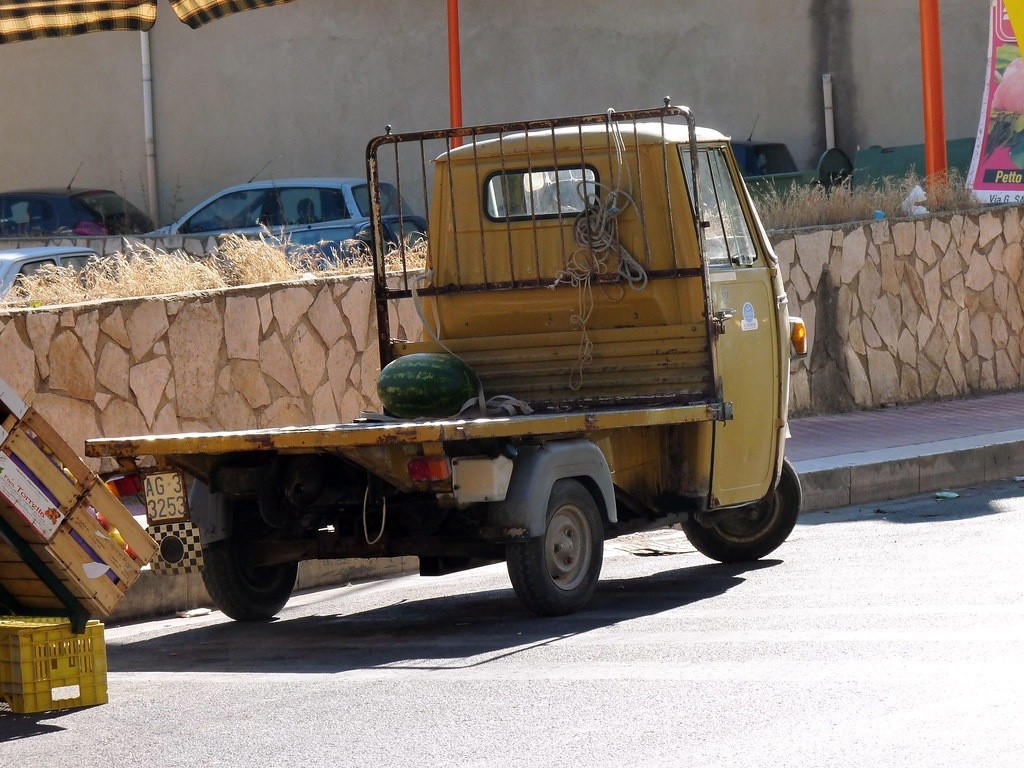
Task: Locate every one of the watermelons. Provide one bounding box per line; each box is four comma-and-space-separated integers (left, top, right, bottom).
375, 353, 474, 418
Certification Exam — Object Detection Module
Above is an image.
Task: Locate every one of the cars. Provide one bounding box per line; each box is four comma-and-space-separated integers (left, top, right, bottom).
0, 246, 109, 304
726, 139, 802, 208
140, 178, 428, 272
0, 187, 156, 238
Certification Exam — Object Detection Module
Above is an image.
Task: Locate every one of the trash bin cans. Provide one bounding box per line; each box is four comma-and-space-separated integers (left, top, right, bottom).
742, 146, 852, 198
854, 137, 976, 189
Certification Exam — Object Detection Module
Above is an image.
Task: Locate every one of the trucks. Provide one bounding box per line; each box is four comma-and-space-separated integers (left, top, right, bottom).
84, 96, 812, 624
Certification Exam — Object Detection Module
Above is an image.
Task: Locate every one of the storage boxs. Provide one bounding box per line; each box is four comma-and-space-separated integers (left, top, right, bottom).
0, 613, 110, 715
0, 375, 162, 620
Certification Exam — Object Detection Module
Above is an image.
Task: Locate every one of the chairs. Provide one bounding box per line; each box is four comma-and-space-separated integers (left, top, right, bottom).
258, 200, 290, 227
28, 200, 48, 231
0, 201, 19, 237
295, 198, 322, 225
